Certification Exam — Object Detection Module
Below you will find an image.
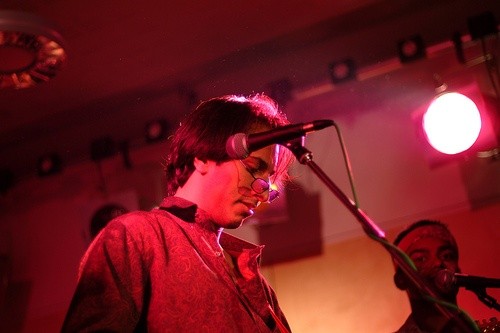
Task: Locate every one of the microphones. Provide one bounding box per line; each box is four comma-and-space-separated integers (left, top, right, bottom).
434, 269, 500, 291
225, 120, 333, 160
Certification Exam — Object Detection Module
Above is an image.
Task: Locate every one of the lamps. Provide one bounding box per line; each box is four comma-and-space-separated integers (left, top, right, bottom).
421, 70, 482, 155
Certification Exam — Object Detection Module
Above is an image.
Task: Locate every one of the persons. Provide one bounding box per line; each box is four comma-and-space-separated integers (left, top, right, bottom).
61, 92, 295, 331
297, 211, 301, 212
378, 219, 486, 331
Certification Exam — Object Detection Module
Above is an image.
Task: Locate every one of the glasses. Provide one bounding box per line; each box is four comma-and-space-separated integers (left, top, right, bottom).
239, 159, 280, 204
